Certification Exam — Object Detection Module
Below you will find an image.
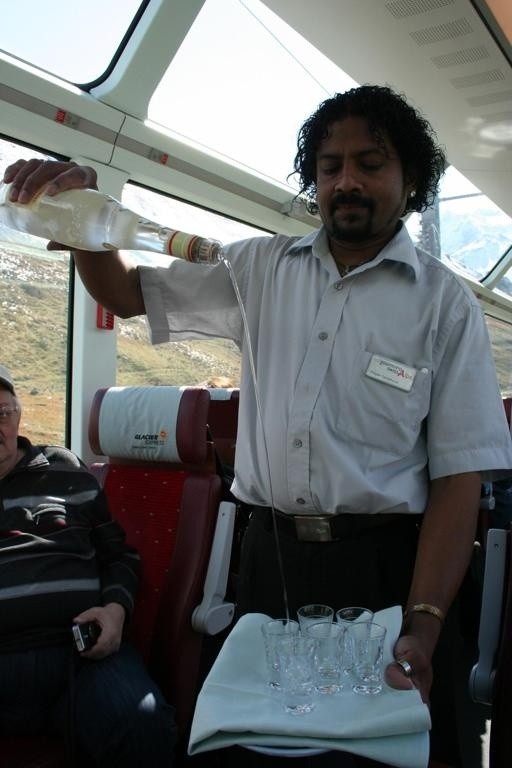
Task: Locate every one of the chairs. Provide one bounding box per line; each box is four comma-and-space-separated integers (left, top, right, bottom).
89, 387, 241, 724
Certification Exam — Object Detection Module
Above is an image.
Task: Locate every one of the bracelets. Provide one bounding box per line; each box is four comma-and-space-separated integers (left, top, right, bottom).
404, 604, 445, 623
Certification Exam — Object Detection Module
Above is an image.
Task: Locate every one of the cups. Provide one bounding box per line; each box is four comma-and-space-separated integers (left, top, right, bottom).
259, 603, 388, 717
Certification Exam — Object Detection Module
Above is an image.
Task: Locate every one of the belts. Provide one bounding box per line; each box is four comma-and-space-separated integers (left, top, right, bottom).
260, 507, 412, 544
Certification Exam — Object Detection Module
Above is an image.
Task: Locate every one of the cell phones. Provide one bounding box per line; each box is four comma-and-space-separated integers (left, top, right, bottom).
72, 620, 101, 653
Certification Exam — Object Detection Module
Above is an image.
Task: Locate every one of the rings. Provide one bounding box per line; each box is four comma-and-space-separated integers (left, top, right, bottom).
397, 660, 413, 676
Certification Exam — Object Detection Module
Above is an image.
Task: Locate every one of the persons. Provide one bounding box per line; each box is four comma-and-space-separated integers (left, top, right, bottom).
0, 378, 180, 768
4, 87, 511, 768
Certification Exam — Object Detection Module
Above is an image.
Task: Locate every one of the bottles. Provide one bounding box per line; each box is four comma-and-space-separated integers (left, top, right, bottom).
0, 176, 224, 268
